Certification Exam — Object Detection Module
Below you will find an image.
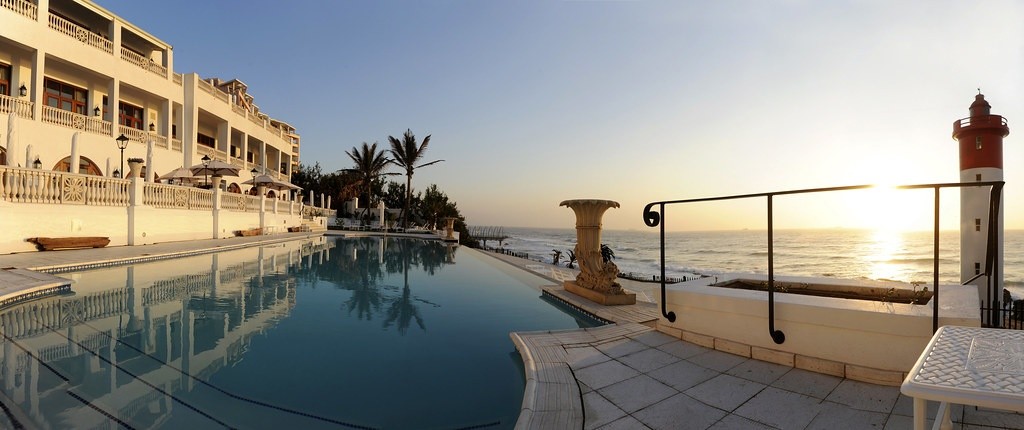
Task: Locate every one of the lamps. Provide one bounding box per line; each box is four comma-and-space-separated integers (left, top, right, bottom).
169, 178, 175, 185
20, 84, 27, 97
94, 106, 101, 117
34, 156, 42, 168
149, 123, 154, 132
113, 169, 121, 178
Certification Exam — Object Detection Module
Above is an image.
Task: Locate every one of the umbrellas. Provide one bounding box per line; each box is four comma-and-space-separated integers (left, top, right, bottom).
189, 161, 240, 188
155, 166, 201, 186
241, 175, 304, 201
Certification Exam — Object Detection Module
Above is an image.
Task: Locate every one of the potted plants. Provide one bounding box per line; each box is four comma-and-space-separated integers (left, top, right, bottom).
297, 194, 304, 202
209, 174, 223, 188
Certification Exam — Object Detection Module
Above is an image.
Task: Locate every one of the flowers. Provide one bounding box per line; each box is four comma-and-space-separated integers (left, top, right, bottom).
125, 157, 144, 163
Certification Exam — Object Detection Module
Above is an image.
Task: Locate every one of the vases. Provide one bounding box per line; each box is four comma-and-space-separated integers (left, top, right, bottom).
128, 163, 143, 178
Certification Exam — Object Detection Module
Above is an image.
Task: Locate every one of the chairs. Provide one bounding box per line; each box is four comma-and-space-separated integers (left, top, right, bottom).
342, 220, 443, 235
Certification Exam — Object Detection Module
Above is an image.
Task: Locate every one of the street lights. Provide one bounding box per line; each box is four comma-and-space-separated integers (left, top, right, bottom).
250, 167, 258, 195
115, 135, 130, 203
201, 155, 212, 189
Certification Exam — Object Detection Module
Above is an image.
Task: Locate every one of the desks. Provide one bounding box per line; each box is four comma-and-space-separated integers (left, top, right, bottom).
900, 325, 1024, 430
